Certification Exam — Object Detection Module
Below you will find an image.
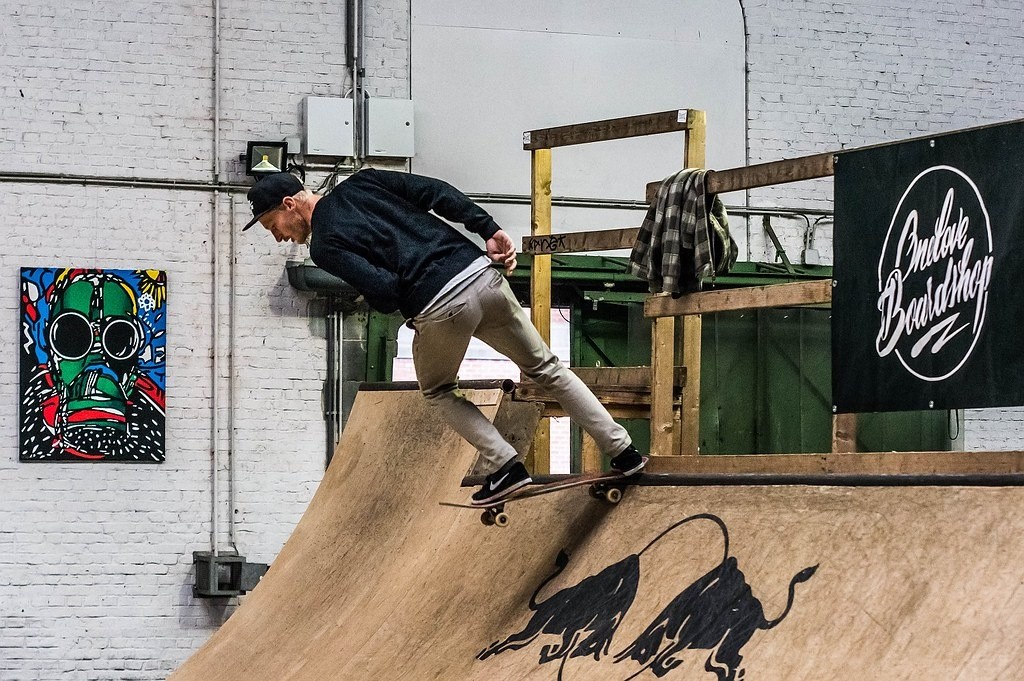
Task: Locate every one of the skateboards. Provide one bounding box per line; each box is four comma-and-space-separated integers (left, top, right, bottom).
437, 453, 650, 527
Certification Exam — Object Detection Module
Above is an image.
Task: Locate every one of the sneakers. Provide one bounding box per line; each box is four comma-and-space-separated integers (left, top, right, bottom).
610, 445, 644, 476
471, 461, 532, 504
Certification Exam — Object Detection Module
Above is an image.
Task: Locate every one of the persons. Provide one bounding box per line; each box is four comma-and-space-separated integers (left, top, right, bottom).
242, 167, 644, 505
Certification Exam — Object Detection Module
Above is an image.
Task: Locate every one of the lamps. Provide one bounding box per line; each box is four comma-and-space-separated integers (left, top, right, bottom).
246, 140, 288, 176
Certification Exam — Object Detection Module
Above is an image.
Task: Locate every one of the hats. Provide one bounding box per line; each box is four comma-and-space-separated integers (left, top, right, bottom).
241, 173, 304, 231
